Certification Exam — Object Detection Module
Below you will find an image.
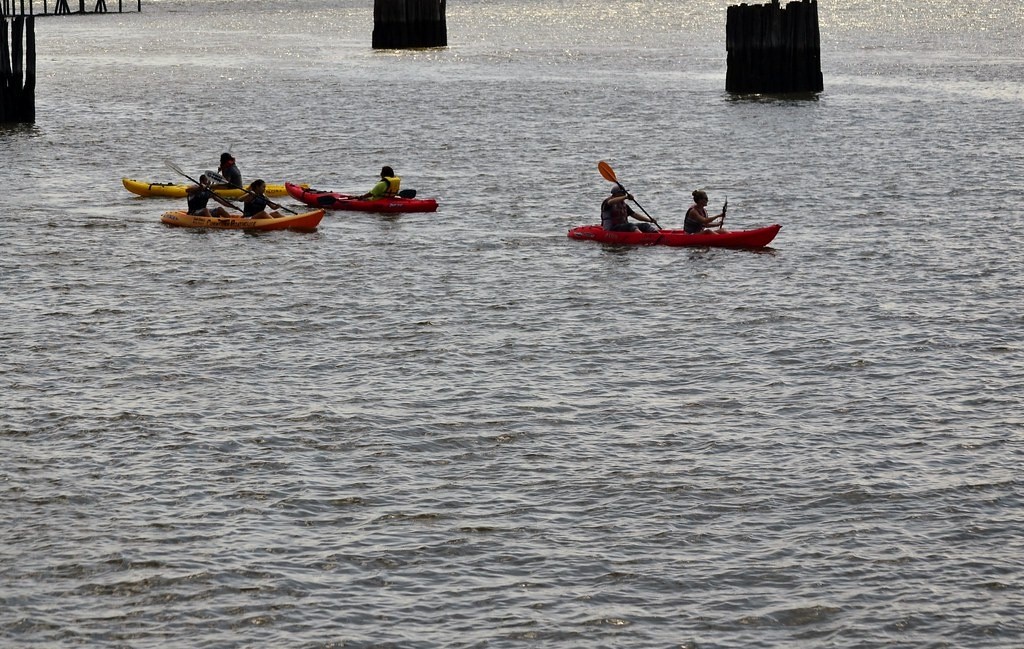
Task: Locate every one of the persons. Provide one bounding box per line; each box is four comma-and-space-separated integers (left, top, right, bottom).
601, 185, 660, 233
186, 174, 232, 218
211, 153, 243, 190
238, 179, 285, 219
684, 189, 732, 235
356, 165, 401, 201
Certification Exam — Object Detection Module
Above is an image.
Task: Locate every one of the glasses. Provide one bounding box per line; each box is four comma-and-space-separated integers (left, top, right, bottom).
701, 197, 708, 200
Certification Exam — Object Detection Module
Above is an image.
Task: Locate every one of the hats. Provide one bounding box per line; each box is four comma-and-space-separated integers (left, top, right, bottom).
611, 185, 630, 194
221, 153, 235, 161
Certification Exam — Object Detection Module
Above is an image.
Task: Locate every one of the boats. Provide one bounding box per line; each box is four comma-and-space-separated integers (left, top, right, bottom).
284, 181, 440, 212
160, 208, 326, 230
567, 223, 785, 249
122, 176, 310, 197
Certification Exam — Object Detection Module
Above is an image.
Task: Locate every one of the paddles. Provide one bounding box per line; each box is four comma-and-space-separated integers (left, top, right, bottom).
596, 158, 661, 231
315, 194, 369, 206
717, 195, 727, 230
208, 144, 234, 186
205, 171, 301, 216
160, 155, 249, 217
